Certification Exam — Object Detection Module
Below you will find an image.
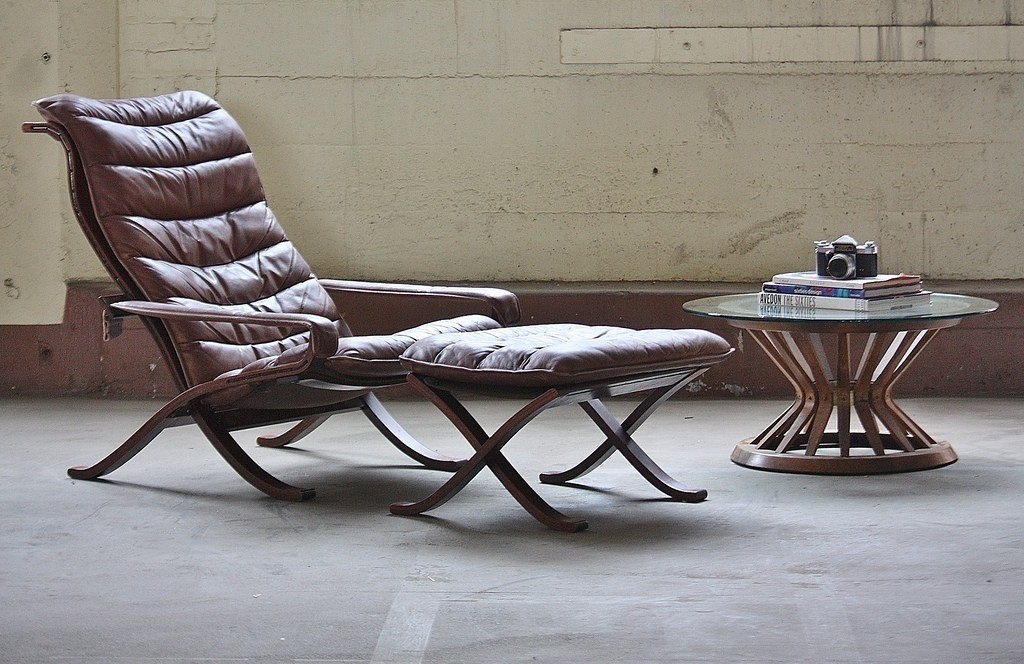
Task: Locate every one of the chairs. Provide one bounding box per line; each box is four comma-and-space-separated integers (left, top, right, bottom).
19, 85, 526, 503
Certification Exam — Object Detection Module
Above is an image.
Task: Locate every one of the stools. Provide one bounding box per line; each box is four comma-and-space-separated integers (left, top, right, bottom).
390, 316, 737, 530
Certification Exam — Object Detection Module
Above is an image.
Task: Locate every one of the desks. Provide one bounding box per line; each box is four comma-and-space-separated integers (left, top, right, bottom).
677, 290, 1005, 481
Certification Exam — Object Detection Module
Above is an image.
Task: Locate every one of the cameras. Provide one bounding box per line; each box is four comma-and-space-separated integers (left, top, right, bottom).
812, 235, 879, 281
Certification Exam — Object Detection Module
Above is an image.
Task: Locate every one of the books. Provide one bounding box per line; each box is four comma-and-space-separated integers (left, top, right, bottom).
758, 270, 936, 312
